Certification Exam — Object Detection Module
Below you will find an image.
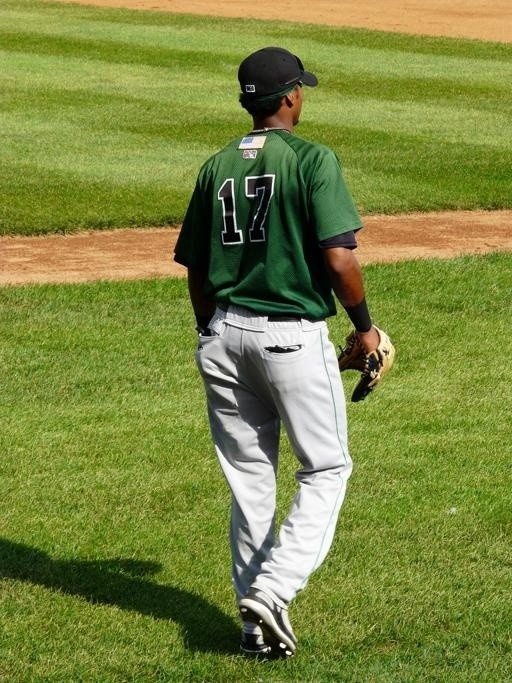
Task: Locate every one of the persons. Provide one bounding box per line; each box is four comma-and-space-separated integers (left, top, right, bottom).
169, 43, 398, 660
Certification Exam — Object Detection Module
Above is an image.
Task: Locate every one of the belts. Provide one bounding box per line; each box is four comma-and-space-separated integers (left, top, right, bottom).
217, 298, 300, 319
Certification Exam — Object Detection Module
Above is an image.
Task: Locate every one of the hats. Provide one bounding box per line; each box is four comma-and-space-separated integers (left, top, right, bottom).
238, 47, 317, 99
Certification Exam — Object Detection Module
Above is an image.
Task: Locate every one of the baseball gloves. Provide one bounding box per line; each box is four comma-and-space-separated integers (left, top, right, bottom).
338, 325, 395, 402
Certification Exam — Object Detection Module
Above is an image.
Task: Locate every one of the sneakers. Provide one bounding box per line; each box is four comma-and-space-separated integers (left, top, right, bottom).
240, 625, 271, 654
238, 583, 297, 659
